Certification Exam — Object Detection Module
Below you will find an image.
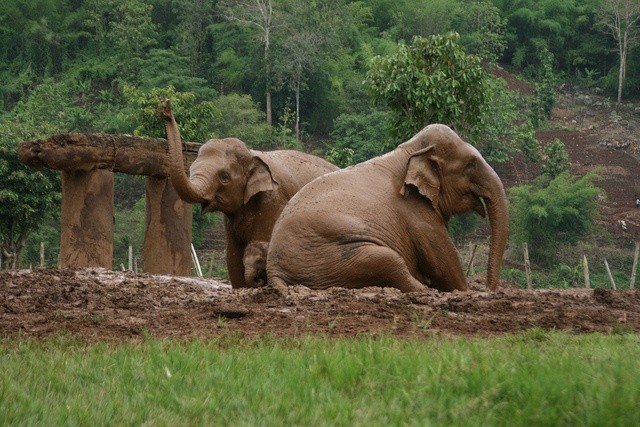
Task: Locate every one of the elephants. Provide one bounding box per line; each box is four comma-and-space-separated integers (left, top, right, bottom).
160, 99, 342, 287
265, 123, 508, 292
243, 240, 269, 286
